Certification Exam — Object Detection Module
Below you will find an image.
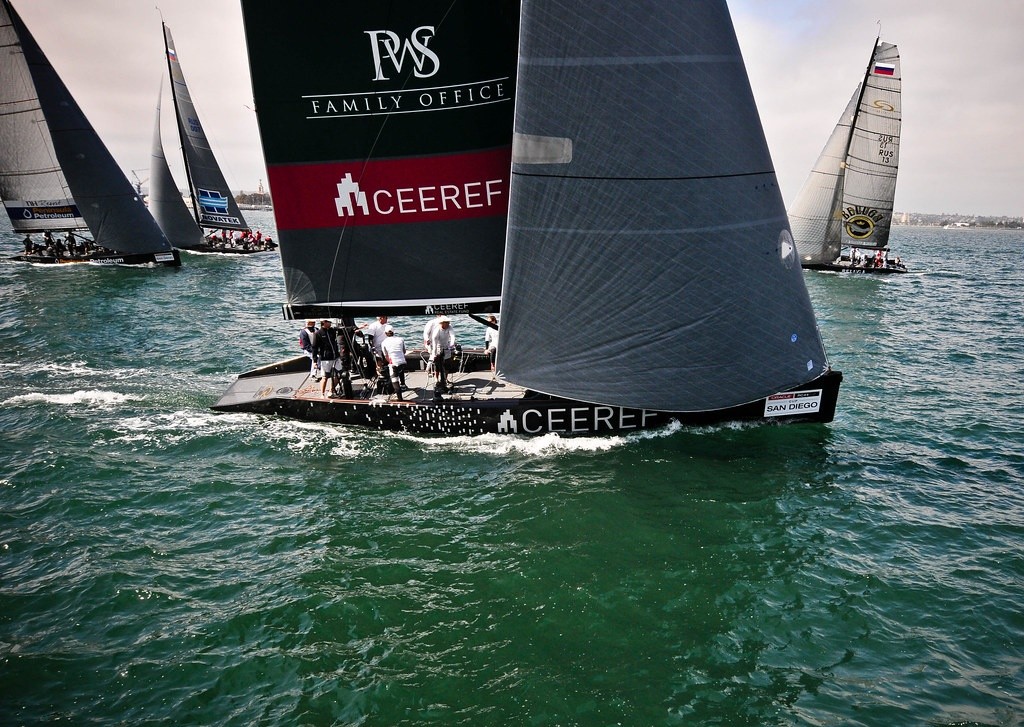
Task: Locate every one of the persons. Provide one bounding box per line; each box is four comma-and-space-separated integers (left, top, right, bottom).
850, 248, 882, 267
484, 316, 499, 379
895, 257, 901, 264
210, 229, 272, 251
299, 316, 455, 400
23, 231, 76, 257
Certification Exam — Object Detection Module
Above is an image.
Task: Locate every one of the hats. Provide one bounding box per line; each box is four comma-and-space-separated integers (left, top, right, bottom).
320, 318, 333, 323
438, 316, 452, 324
385, 325, 395, 333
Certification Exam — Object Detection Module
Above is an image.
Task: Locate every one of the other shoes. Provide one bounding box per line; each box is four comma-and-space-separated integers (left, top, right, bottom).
322, 395, 326, 398
310, 377, 318, 382
400, 384, 406, 389
327, 392, 336, 396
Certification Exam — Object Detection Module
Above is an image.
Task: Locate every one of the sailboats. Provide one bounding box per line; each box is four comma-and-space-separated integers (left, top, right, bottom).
780, 19, 912, 277
0, 0, 183, 269
207, 0, 846, 445
133, 6, 279, 256
